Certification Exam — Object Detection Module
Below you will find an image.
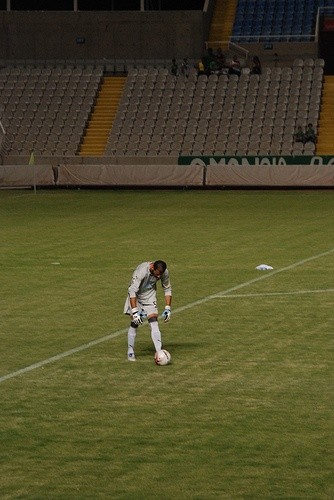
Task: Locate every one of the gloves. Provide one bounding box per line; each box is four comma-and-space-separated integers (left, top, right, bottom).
132, 307, 143, 325
162, 305, 171, 322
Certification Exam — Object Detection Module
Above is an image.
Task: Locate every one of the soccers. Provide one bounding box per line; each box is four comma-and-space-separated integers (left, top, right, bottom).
154, 349, 171, 366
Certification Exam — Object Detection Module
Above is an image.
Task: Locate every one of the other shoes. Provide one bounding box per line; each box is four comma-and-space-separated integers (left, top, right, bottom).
128, 351, 136, 361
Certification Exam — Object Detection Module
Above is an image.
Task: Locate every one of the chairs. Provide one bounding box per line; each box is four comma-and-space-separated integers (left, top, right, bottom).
0, 58, 324, 156
231, 0, 334, 42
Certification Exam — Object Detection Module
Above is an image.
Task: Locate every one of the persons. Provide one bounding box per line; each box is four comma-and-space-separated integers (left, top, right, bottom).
295, 123, 316, 143
228, 56, 241, 76
180, 58, 191, 77
197, 48, 226, 78
249, 56, 261, 76
171, 58, 178, 76
124, 260, 173, 361
